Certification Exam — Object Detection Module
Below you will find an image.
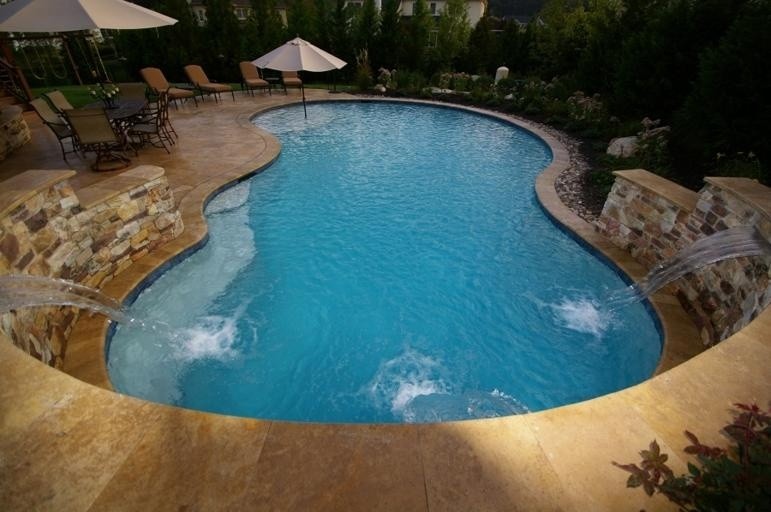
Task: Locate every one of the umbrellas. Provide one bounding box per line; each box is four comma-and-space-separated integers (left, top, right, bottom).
249, 35, 349, 119
1, 0, 180, 89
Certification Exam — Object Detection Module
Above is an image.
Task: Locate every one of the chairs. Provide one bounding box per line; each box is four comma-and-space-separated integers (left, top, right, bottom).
239, 60, 304, 98
139, 65, 236, 111
28, 82, 181, 172
